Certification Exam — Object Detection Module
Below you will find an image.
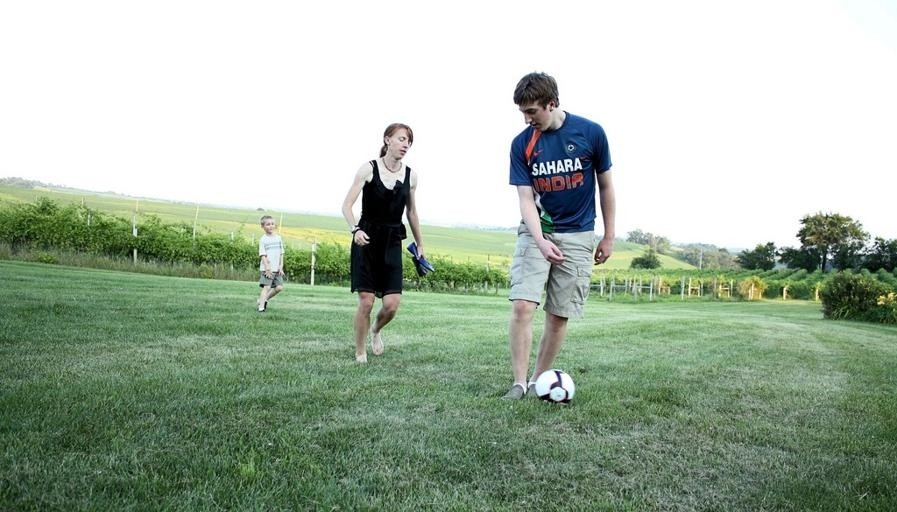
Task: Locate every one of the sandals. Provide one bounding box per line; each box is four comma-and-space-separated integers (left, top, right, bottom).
407, 242, 434, 277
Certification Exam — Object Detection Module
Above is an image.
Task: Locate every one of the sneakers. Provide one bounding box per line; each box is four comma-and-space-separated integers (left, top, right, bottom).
501, 384, 536, 399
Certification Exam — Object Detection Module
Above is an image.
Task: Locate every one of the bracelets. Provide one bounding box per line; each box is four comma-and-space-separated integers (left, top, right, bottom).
350, 225, 361, 234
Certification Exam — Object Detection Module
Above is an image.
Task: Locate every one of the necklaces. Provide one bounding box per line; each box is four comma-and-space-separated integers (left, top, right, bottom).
381, 156, 402, 173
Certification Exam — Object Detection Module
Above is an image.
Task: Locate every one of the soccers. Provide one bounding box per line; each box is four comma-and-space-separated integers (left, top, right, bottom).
535, 368, 575, 405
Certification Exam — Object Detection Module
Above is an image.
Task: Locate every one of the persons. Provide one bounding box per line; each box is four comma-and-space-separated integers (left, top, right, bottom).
342, 124, 426, 364
500, 73, 615, 403
256, 215, 285, 312
